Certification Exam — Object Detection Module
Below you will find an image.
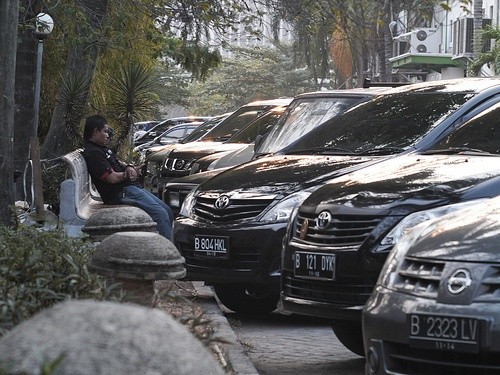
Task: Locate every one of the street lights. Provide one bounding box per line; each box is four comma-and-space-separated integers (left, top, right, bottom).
31, 13, 53, 161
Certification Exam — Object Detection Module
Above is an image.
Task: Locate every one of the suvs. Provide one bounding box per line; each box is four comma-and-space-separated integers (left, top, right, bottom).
126, 75, 500, 375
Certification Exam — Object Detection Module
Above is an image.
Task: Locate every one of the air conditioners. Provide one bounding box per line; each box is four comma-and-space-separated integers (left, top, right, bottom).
409, 27, 442, 55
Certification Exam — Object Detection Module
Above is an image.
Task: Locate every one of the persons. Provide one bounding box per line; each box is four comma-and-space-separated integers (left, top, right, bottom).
134, 124, 147, 140
84, 114, 174, 241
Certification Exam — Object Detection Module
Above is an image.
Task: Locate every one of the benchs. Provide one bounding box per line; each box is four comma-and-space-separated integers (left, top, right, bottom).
57, 147, 104, 239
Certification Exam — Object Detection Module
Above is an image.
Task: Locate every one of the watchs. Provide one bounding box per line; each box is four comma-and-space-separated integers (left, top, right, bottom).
125, 163, 134, 168
